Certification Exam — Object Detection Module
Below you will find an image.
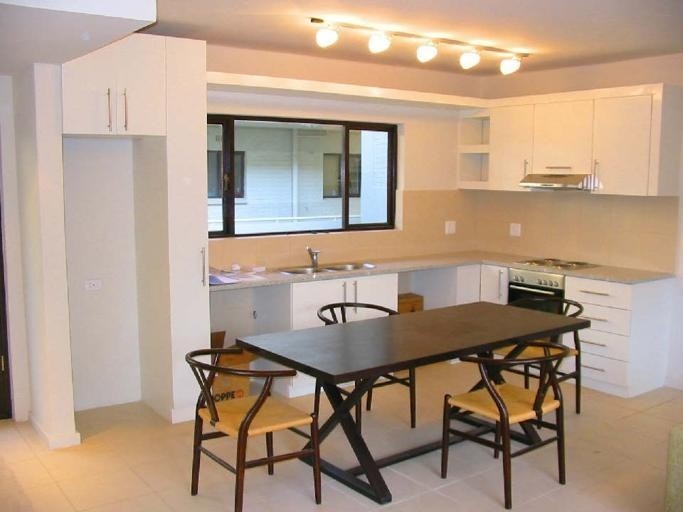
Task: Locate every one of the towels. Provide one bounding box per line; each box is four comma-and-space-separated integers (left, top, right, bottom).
478, 266, 500, 305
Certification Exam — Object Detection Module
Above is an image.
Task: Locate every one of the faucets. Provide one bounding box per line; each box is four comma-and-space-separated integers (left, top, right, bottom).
305, 245, 319, 268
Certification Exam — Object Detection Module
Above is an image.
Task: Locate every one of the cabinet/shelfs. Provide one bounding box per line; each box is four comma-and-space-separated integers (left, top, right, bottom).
406, 262, 508, 311
592, 82, 682, 198
251, 273, 403, 395
490, 104, 532, 190
560, 269, 674, 398
60, 35, 165, 136
534, 100, 593, 175
456, 108, 488, 190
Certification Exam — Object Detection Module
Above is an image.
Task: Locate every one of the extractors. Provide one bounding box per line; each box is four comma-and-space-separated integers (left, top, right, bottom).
518, 174, 593, 191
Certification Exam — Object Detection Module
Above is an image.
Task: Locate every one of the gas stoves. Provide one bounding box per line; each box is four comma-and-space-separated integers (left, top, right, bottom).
509, 259, 597, 290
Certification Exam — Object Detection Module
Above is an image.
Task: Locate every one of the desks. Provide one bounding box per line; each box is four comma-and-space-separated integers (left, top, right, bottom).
229, 297, 593, 506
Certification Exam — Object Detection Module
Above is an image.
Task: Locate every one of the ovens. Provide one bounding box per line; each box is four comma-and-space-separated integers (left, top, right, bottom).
507, 282, 565, 346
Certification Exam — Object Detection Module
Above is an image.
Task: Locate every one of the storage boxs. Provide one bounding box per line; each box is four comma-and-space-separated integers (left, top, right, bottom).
210, 328, 261, 405
397, 292, 425, 315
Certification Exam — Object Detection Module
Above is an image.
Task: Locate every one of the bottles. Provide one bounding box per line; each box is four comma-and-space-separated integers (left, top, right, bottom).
236, 188, 242, 197
331, 189, 337, 196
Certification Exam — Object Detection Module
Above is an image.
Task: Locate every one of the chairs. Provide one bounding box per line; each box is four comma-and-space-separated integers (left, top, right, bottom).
436, 336, 571, 510
181, 345, 325, 511
479, 296, 583, 417
314, 299, 422, 434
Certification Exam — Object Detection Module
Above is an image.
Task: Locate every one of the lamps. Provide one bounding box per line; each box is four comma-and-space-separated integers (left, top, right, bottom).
309, 15, 532, 74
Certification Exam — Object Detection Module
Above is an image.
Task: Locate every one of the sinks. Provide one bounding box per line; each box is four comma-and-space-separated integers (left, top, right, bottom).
324, 261, 379, 272
281, 267, 331, 276
